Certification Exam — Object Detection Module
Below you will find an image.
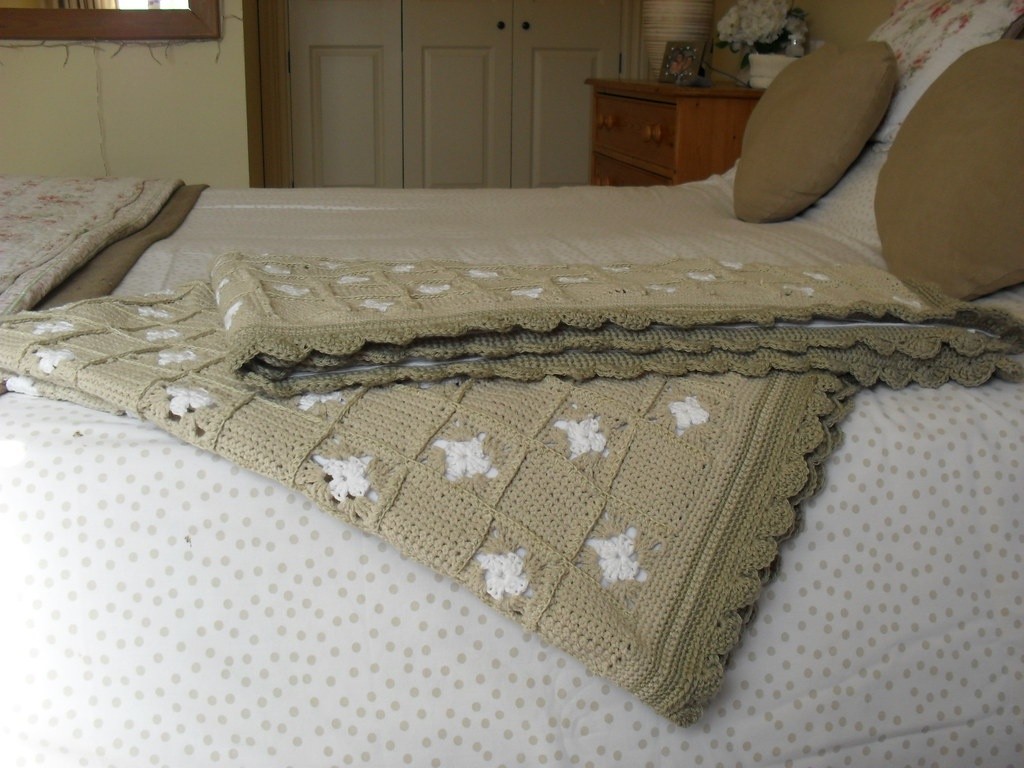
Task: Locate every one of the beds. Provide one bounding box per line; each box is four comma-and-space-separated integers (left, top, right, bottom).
0, 141, 1024, 768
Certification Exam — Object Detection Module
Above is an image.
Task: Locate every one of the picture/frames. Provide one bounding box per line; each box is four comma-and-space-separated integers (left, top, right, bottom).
657, 41, 707, 84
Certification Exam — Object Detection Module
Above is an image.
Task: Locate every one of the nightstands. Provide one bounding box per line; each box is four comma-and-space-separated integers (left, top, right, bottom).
585, 77, 766, 188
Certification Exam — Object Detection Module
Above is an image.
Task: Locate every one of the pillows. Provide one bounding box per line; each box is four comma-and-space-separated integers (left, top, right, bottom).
731, 38, 896, 222
874, 42, 1024, 300
866, 0, 1024, 144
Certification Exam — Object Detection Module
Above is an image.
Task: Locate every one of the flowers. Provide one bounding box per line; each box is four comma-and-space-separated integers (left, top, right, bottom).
716, 1, 808, 55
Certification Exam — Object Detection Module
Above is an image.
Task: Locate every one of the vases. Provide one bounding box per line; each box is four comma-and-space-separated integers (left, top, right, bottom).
747, 53, 800, 88
